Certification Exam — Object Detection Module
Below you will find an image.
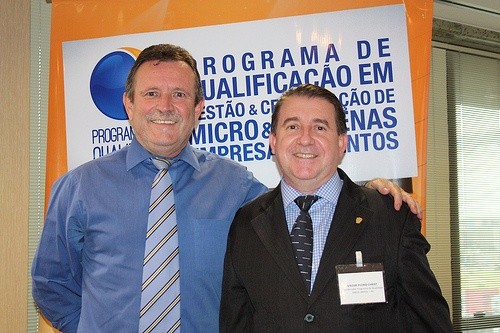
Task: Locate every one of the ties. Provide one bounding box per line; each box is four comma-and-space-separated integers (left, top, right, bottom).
289, 196, 322, 295
137, 157, 182, 333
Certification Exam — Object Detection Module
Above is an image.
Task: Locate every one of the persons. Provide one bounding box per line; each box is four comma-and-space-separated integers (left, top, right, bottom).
219, 83, 455, 333
30, 42, 422, 332
